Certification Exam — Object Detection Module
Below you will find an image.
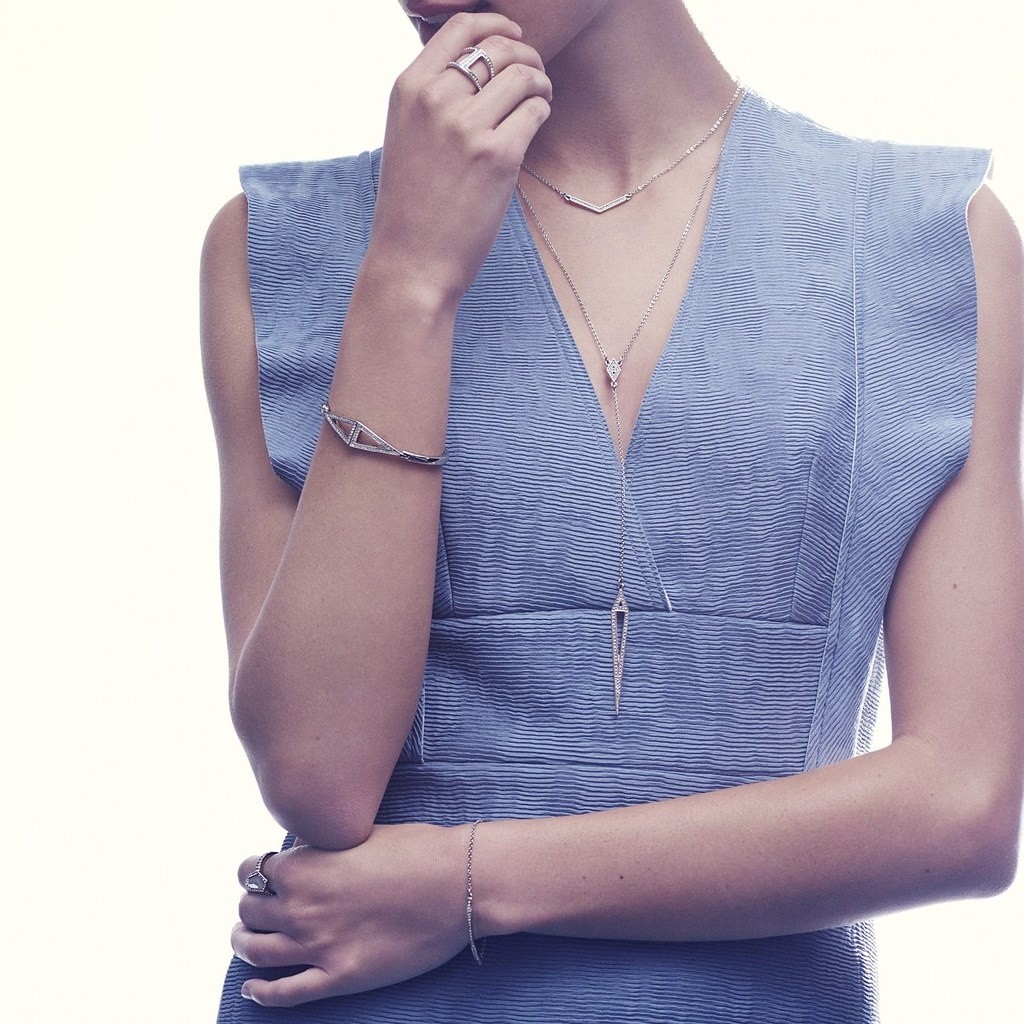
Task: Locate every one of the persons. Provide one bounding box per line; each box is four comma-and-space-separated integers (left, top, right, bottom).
198, 1, 1024, 1024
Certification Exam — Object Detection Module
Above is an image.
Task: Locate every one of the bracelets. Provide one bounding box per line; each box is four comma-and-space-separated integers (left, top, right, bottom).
465, 817, 489, 967
318, 401, 448, 467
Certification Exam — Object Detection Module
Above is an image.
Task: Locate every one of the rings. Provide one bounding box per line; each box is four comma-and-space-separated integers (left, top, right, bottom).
446, 47, 497, 95
246, 852, 277, 898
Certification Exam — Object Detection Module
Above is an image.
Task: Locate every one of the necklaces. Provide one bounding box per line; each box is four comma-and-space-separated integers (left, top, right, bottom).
514, 73, 747, 716
